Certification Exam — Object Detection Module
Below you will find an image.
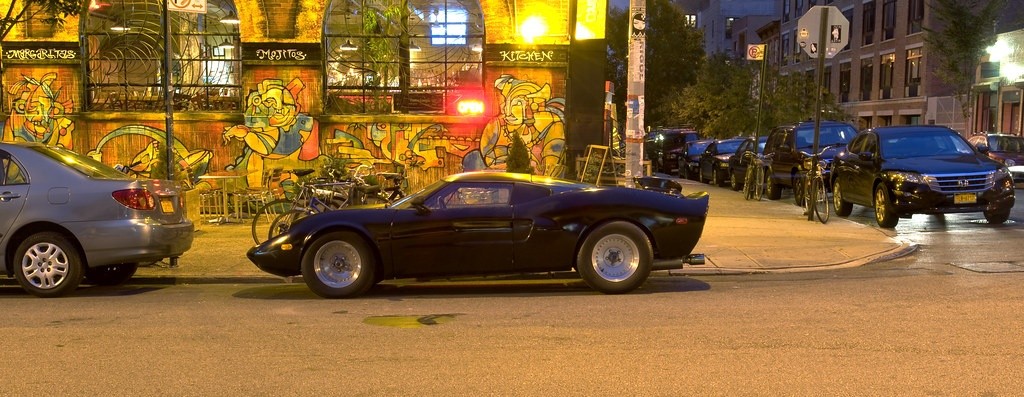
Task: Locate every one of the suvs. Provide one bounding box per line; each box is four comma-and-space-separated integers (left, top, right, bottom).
762, 121, 861, 206
647, 129, 701, 173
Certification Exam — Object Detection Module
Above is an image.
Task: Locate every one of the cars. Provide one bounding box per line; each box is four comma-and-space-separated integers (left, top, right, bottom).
677, 140, 716, 179
728, 136, 769, 190
0, 140, 195, 297
965, 133, 1024, 182
699, 138, 752, 186
830, 124, 1016, 228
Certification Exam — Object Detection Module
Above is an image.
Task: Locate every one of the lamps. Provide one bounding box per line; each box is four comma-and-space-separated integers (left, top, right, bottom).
410, 17, 421, 51
340, 18, 357, 51
219, 10, 240, 23
218, 38, 234, 48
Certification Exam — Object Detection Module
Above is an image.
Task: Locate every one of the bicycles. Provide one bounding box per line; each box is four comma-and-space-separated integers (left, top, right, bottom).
799, 145, 829, 223
741, 151, 775, 200
252, 167, 405, 246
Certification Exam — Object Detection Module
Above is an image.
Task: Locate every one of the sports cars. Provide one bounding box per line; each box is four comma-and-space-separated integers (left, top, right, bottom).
247, 173, 710, 298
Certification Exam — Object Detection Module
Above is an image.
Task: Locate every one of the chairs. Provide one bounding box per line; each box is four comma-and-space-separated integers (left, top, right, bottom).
233, 166, 285, 224
186, 167, 232, 225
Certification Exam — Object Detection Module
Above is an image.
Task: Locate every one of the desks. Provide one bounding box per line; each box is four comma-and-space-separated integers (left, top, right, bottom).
197, 169, 259, 226
